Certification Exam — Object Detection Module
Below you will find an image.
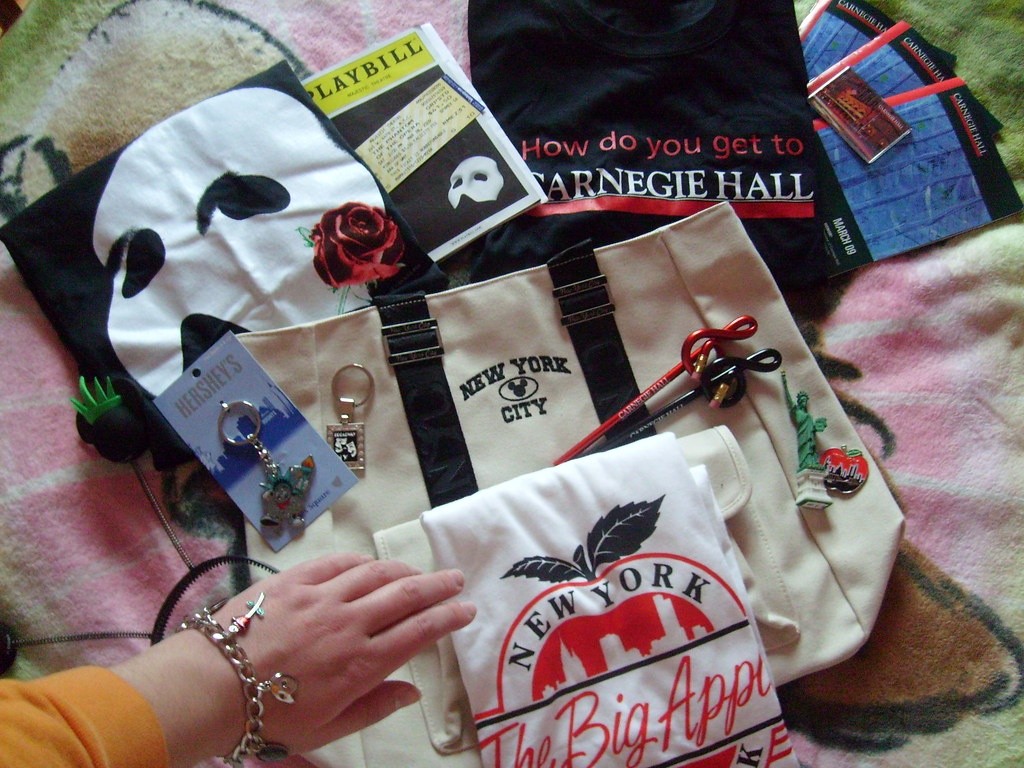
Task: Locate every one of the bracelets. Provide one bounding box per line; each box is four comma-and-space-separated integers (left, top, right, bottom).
176, 591, 298, 768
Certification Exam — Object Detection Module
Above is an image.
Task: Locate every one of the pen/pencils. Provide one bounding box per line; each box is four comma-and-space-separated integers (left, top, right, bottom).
551, 314, 782, 470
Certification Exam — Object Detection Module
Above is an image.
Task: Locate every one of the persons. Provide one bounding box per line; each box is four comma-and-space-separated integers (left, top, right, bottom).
1, 554, 481, 768
781, 371, 827, 471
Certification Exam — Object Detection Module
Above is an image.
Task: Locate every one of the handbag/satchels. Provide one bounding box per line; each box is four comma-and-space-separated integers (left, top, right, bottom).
152, 200, 907, 768
